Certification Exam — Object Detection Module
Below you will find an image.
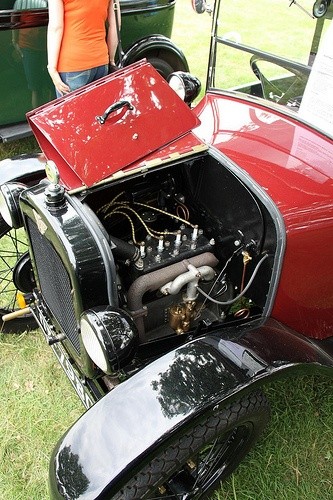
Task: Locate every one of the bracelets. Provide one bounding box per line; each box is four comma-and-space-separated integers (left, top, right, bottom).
46, 65, 58, 69
109, 59, 115, 63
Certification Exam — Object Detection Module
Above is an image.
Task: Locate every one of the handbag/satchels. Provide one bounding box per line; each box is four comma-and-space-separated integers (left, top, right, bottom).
108, 65, 119, 74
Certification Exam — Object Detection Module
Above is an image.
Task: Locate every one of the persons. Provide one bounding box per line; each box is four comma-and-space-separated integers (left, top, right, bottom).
11, 26, 55, 110
46, 0, 119, 100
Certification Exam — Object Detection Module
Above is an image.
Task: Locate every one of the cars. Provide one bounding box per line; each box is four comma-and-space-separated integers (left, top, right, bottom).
0, 2, 333, 500
